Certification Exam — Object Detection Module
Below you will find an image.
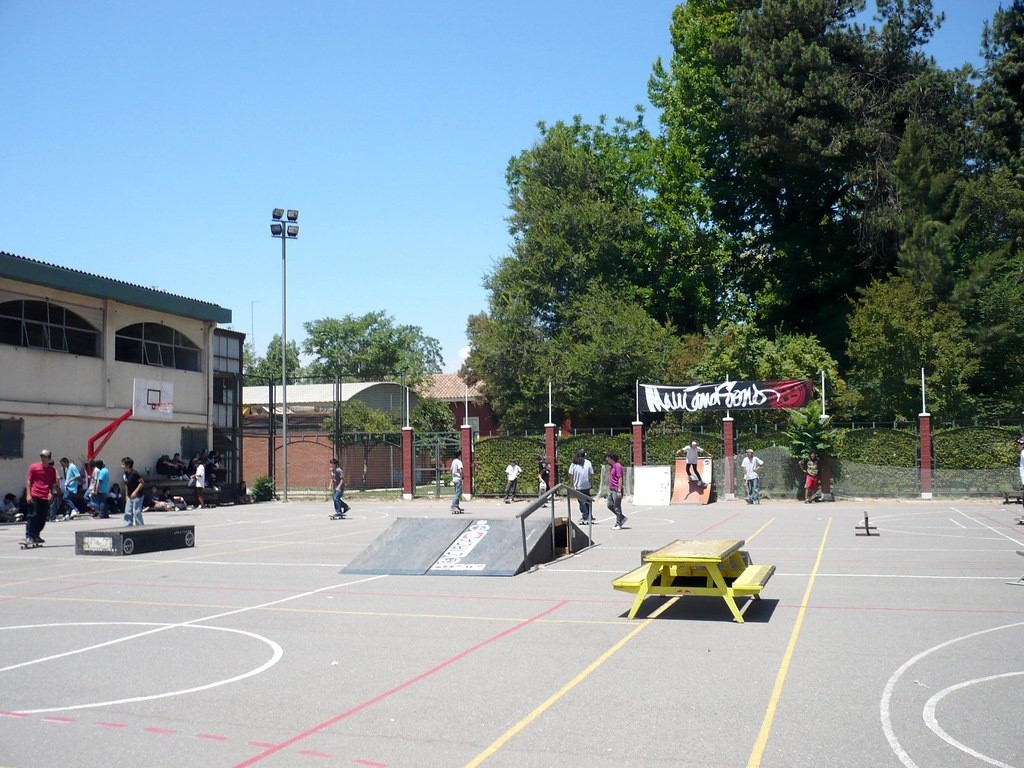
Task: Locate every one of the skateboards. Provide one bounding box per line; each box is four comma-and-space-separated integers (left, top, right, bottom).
578, 518, 596, 525
328, 514, 347, 520
503, 499, 510, 504
690, 480, 708, 488
18, 542, 44, 549
449, 509, 464, 514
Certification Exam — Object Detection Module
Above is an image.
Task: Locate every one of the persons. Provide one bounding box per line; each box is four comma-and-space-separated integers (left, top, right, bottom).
677, 442, 713, 486
25, 449, 56, 544
798, 451, 819, 504
0, 457, 126, 523
569, 449, 595, 521
140, 449, 254, 512
740, 449, 764, 505
607, 451, 628, 530
534, 455, 552, 508
121, 457, 145, 528
504, 460, 523, 501
330, 459, 351, 516
1016, 437, 1024, 520
450, 450, 464, 513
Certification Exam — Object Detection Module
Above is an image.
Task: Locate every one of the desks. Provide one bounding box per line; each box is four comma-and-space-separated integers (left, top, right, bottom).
627, 538, 760, 623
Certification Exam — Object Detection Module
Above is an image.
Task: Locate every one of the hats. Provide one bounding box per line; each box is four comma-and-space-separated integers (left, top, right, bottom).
578, 450, 587, 458
40, 450, 50, 456
746, 449, 753, 452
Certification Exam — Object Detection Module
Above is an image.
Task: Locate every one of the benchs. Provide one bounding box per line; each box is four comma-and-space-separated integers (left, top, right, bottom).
732, 564, 776, 590
611, 563, 664, 587
141, 473, 219, 507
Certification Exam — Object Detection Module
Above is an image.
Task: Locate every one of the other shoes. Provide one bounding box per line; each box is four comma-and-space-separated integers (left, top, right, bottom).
580, 518, 587, 522
179, 476, 182, 480
335, 512, 341, 515
457, 507, 464, 511
183, 475, 189, 480
451, 508, 459, 511
343, 507, 351, 513
175, 476, 178, 479
592, 517, 595, 520
71, 510, 80, 517
170, 476, 173, 480
26, 538, 33, 544
34, 537, 44, 542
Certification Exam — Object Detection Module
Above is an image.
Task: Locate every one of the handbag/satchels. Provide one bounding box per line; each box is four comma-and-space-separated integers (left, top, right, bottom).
188, 478, 196, 487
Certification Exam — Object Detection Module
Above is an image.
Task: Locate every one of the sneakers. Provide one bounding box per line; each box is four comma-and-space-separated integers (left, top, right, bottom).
620, 517, 628, 525
612, 524, 621, 529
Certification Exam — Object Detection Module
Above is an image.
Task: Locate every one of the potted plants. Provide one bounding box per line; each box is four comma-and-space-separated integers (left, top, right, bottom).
252, 476, 274, 501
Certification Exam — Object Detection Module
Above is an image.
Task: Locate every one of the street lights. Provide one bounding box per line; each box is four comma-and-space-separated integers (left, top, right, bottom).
268, 208, 300, 504
